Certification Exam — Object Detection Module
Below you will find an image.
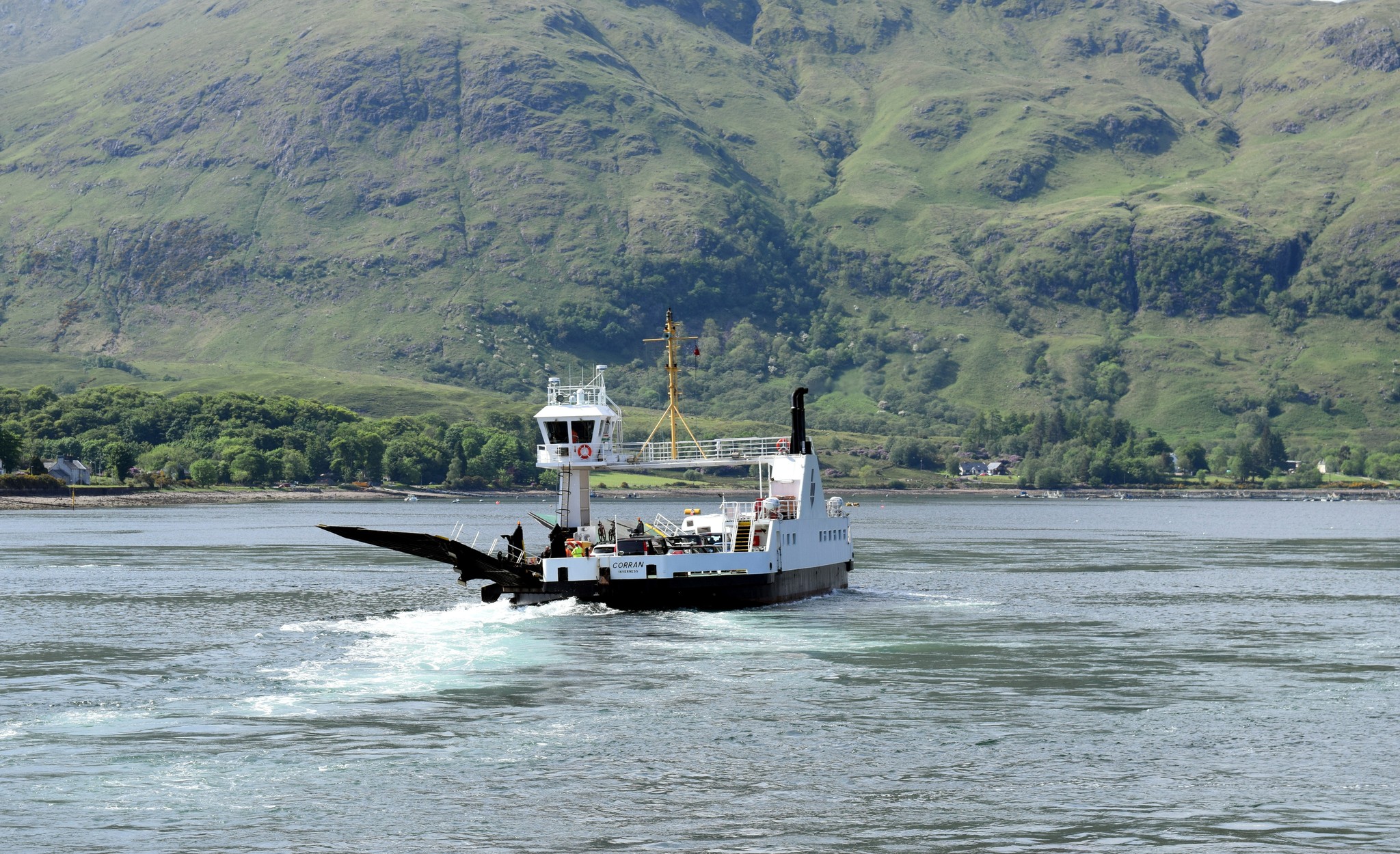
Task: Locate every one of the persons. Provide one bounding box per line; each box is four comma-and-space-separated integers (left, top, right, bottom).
634, 522, 643, 533
542, 543, 594, 558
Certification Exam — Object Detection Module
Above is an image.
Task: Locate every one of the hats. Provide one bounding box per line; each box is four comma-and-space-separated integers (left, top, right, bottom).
573, 543, 578, 546
565, 544, 568, 546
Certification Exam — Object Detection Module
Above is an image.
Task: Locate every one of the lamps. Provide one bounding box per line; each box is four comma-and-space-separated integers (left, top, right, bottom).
556, 394, 564, 402
627, 457, 635, 462
731, 452, 740, 459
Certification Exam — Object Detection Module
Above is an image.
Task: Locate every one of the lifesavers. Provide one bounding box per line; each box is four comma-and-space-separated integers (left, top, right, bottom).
577, 445, 592, 459
777, 439, 790, 452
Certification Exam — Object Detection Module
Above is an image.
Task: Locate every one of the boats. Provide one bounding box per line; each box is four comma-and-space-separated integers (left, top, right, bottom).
317, 307, 857, 614
404, 494, 418, 501
589, 490, 603, 498
626, 493, 643, 498
846, 502, 860, 507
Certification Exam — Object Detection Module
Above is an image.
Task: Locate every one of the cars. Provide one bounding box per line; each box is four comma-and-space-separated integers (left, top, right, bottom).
279, 483, 291, 488
290, 484, 296, 487
429, 482, 437, 485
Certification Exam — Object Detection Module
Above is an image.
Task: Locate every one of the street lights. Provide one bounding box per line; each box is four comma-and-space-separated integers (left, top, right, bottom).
31, 466, 33, 474
99, 453, 103, 485
78, 457, 82, 464
3, 465, 5, 474
